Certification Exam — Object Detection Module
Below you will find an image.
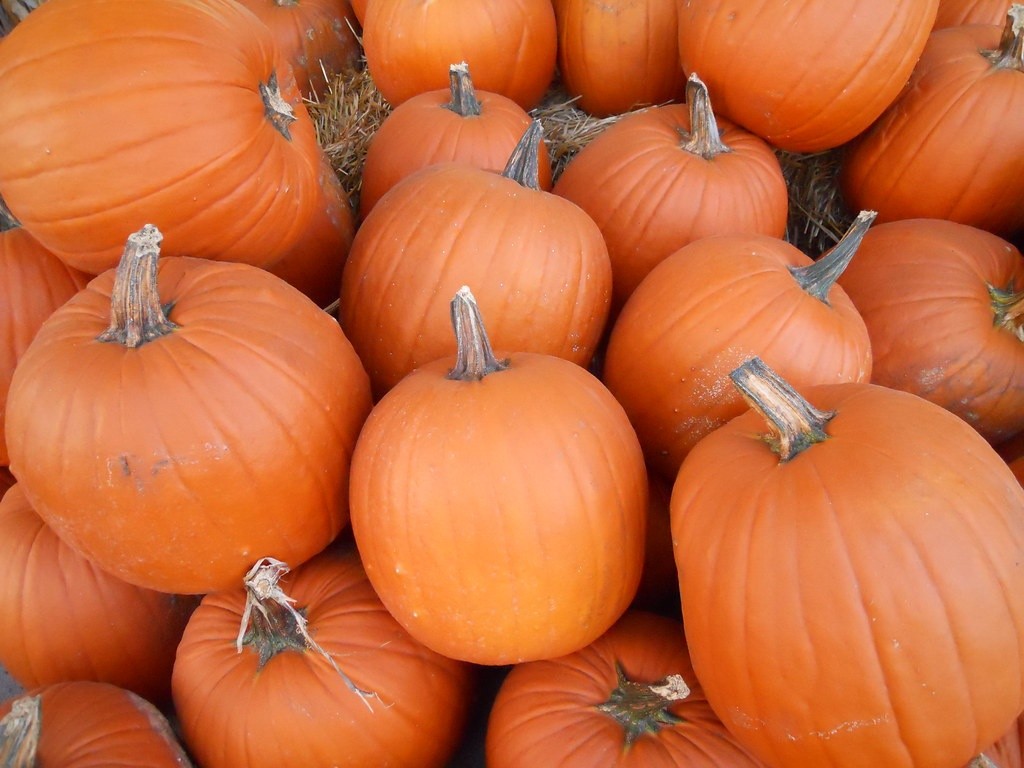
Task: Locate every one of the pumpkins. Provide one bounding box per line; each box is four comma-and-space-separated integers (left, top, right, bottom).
0, 0, 1024, 768
